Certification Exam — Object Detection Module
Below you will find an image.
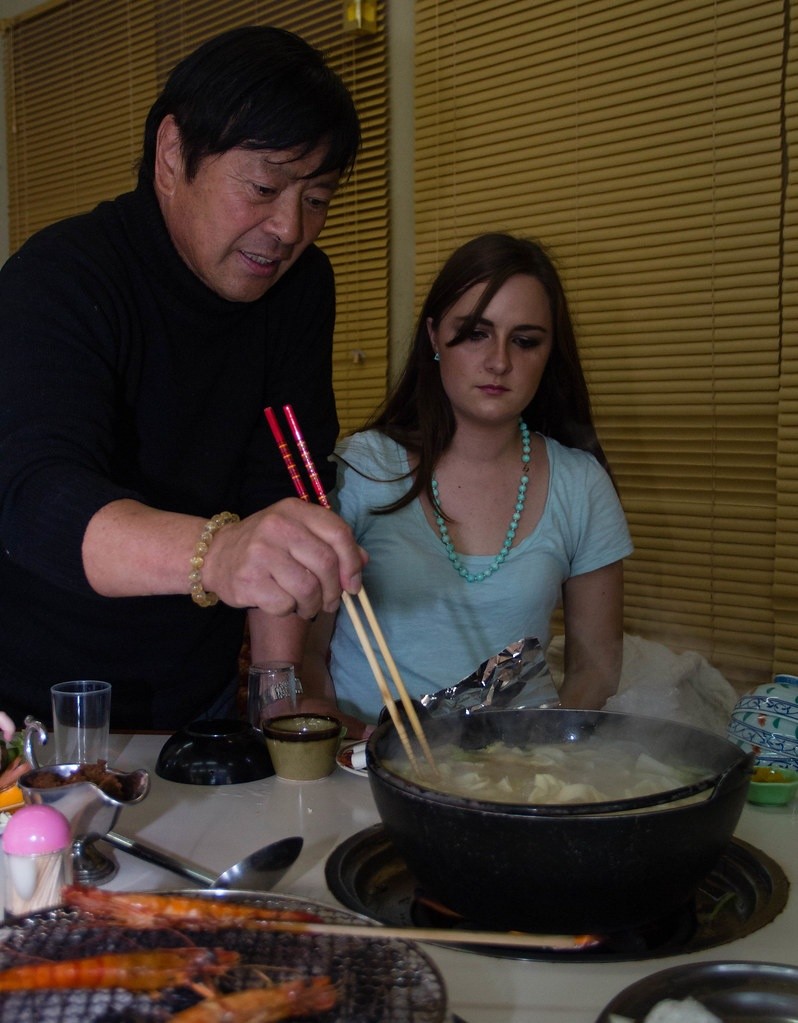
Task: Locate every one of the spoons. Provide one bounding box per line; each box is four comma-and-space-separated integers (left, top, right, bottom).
101, 830, 306, 893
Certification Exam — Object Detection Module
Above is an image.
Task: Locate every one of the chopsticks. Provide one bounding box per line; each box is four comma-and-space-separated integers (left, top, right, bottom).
264, 404, 440, 779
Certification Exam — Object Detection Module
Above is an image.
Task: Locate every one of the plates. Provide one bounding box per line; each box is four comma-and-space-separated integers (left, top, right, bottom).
594, 959, 798, 1023
336, 737, 369, 779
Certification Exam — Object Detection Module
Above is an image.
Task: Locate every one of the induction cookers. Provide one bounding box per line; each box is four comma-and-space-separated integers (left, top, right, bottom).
322, 817, 792, 966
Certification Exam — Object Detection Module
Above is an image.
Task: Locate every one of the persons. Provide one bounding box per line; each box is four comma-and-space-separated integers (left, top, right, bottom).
297, 231, 634, 745
3, 25, 378, 748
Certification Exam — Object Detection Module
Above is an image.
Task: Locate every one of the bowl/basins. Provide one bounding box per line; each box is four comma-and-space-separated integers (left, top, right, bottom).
727, 673, 798, 725
261, 713, 341, 782
747, 764, 798, 806
154, 719, 277, 787
727, 736, 797, 772
725, 711, 798, 759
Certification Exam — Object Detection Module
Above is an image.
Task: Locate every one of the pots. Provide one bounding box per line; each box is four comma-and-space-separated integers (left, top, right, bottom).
364, 697, 760, 918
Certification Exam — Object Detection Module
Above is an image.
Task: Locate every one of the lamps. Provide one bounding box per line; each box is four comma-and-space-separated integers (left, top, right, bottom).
342, 0, 378, 36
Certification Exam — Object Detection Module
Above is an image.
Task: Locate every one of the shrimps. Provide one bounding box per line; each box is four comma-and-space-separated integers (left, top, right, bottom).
165, 976, 350, 1022
1, 926, 303, 1002
59, 882, 327, 948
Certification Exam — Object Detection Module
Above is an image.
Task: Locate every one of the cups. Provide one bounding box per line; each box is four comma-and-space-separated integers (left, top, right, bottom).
247, 660, 297, 732
49, 679, 112, 765
19, 712, 150, 888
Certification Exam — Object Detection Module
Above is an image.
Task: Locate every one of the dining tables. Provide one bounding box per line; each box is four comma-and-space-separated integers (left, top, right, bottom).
0, 723, 798, 1023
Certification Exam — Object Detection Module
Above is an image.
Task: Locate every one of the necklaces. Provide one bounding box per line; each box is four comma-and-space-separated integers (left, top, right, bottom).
430, 416, 533, 583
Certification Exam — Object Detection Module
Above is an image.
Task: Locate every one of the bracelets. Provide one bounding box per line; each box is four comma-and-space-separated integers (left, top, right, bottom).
188, 509, 239, 611
